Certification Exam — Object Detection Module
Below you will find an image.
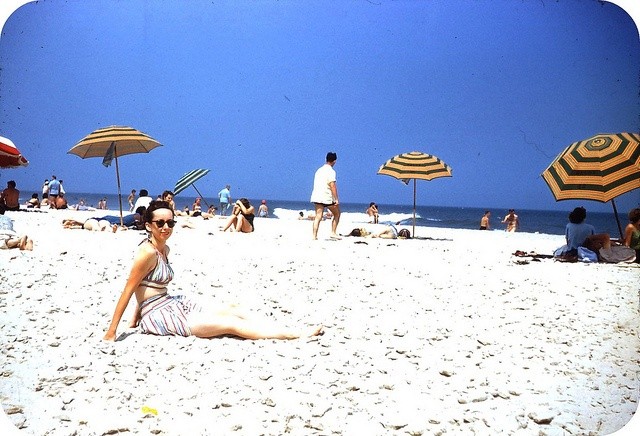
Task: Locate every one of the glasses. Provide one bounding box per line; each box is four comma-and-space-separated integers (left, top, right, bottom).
150, 218, 178, 228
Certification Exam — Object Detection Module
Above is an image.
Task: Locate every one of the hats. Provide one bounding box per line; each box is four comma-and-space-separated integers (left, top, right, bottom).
196, 196, 202, 200
262, 200, 266, 204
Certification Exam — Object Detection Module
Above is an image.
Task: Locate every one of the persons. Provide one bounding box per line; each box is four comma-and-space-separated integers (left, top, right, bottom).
501, 209, 520, 233
218, 184, 232, 216
161, 191, 180, 209
101, 206, 146, 225
25, 192, 40, 211
0, 203, 34, 250
192, 198, 204, 212
623, 206, 640, 255
480, 211, 492, 230
207, 205, 217, 216
298, 211, 305, 222
368, 202, 381, 226
582, 234, 640, 263
65, 217, 119, 234
104, 201, 330, 343
125, 188, 135, 213
309, 151, 342, 241
224, 198, 256, 233
257, 199, 269, 218
137, 188, 156, 208
0, 181, 20, 214
348, 226, 397, 239
41, 176, 108, 211
552, 207, 596, 262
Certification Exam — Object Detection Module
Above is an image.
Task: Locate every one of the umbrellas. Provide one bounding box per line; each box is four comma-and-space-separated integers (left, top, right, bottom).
537, 132, 640, 246
174, 166, 212, 208
67, 123, 163, 227
0, 132, 29, 169
375, 150, 452, 238
